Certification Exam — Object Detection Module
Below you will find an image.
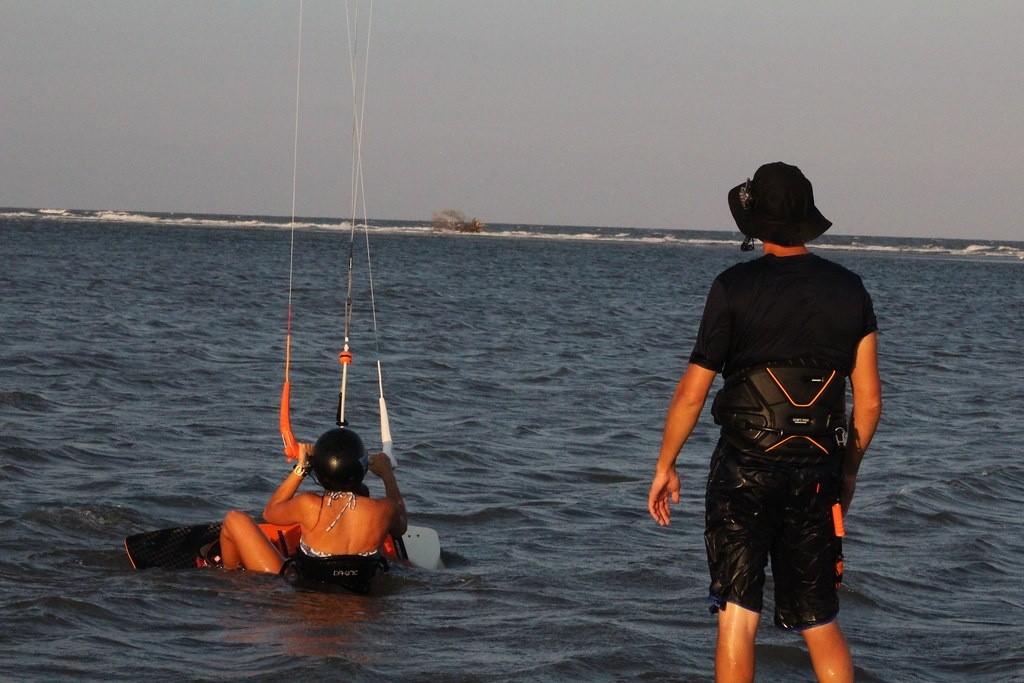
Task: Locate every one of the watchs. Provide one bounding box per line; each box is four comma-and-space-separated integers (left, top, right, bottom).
293, 465, 306, 476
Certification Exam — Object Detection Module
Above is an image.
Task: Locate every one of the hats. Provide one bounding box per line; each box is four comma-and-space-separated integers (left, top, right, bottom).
728, 161, 836, 246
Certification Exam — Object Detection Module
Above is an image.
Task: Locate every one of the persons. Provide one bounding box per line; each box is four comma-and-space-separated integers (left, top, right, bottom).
219, 428, 407, 592
649, 162, 882, 683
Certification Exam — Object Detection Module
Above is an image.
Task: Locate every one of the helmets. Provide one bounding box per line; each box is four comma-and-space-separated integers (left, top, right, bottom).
309, 426, 367, 491
